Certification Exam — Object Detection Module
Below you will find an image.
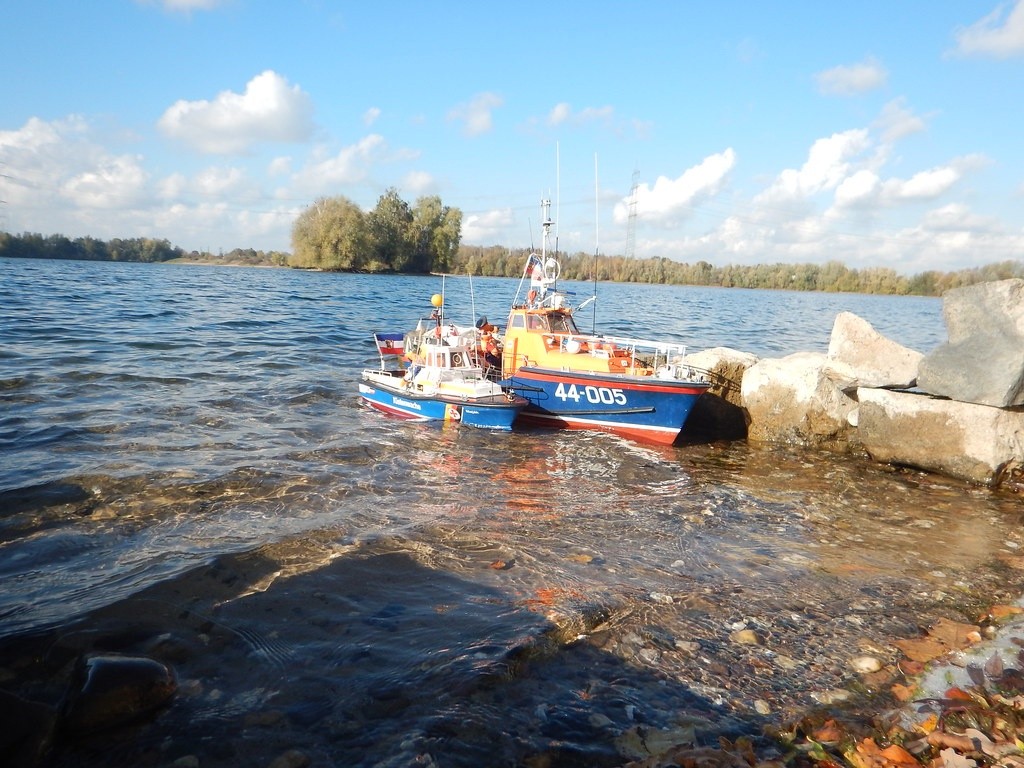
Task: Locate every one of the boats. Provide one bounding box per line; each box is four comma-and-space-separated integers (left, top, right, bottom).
354, 141, 713, 447
